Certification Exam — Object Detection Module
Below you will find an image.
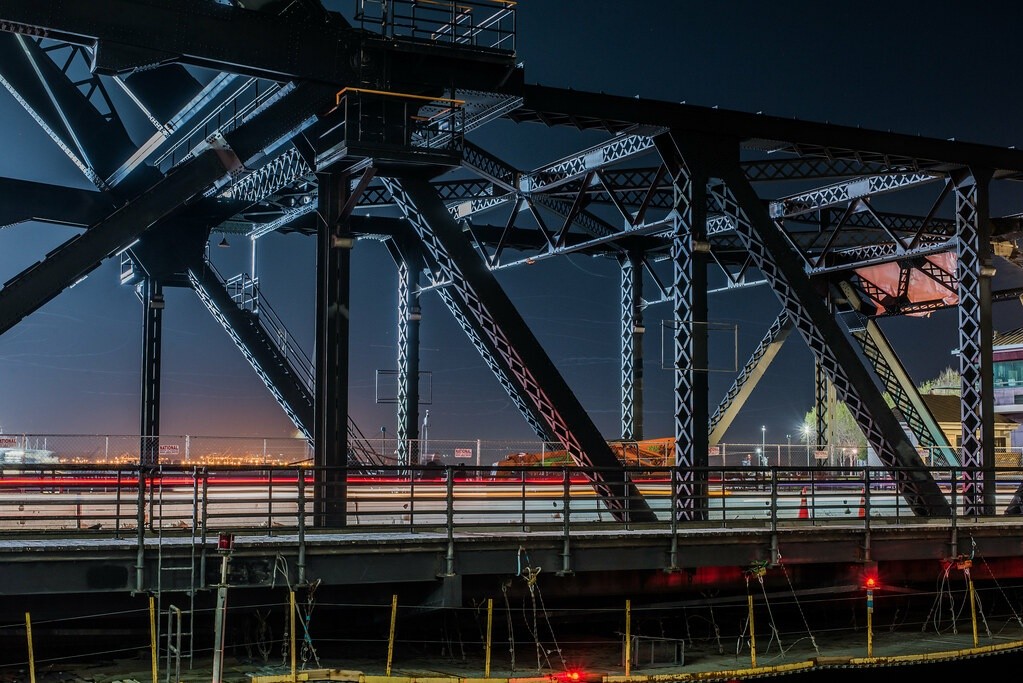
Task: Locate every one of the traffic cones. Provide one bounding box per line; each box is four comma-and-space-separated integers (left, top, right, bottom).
859, 487, 869, 514
798, 487, 812, 520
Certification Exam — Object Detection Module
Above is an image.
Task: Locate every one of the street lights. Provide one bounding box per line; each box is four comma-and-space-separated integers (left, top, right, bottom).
786, 435, 792, 466
761, 425, 767, 458
211, 529, 236, 683
757, 448, 761, 466
380, 426, 386, 464
805, 426, 811, 467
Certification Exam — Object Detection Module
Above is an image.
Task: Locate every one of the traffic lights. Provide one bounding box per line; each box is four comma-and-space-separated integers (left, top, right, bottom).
864, 564, 879, 589
566, 668, 585, 681
